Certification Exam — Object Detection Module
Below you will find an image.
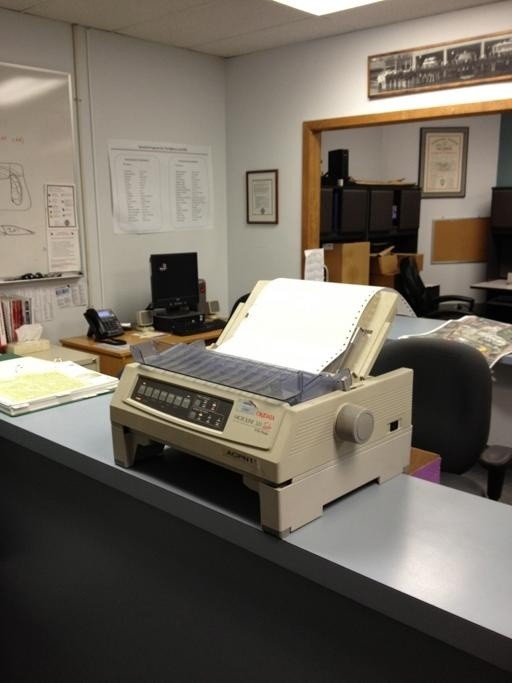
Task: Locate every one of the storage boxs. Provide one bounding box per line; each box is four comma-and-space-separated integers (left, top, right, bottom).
322, 238, 424, 288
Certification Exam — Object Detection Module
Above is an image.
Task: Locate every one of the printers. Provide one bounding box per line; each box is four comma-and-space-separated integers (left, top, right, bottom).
109, 280, 415, 538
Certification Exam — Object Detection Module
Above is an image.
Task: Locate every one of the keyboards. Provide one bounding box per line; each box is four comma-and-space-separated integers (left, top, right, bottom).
172, 319, 227, 335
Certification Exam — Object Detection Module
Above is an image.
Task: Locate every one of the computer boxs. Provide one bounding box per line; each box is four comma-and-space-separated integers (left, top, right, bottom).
153, 310, 205, 332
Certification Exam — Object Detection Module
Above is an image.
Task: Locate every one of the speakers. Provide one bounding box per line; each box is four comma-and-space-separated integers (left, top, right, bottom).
206, 300, 220, 320
137, 310, 155, 331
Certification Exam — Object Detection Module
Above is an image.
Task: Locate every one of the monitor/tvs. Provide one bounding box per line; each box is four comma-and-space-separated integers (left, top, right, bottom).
150, 252, 199, 314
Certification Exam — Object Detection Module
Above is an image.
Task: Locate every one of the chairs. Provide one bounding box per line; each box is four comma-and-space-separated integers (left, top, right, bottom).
399, 257, 475, 320
369, 338, 511, 504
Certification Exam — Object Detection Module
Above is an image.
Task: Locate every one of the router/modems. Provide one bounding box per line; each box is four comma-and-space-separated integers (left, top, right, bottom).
198, 279, 206, 314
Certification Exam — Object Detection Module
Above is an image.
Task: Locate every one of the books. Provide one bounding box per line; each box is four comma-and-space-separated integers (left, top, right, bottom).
0, 356, 120, 417
0, 295, 34, 353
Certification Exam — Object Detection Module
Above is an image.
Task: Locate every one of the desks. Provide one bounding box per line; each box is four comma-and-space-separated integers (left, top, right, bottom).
60, 316, 226, 377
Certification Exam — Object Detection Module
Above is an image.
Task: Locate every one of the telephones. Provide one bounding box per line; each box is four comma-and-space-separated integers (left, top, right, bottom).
84, 307, 124, 340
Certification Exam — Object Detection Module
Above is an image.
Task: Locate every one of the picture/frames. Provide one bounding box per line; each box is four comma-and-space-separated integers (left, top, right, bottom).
369, 31, 511, 100
246, 169, 278, 223
417, 127, 469, 198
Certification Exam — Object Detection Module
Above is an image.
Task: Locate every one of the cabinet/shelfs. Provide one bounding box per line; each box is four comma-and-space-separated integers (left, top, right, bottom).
319, 181, 422, 254
